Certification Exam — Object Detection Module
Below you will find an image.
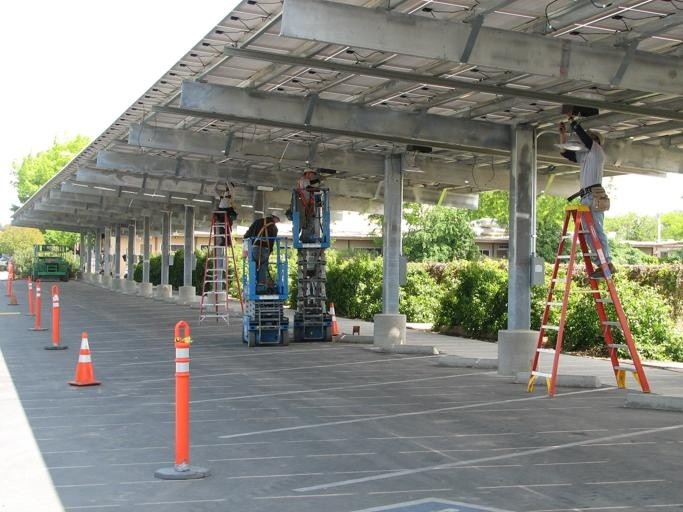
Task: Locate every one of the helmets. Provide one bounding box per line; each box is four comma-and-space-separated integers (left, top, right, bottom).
272, 210, 283, 223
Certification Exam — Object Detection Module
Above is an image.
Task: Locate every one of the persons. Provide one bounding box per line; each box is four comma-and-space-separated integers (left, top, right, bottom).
242, 210, 283, 293
558, 109, 617, 278
296, 167, 319, 242
214, 175, 239, 246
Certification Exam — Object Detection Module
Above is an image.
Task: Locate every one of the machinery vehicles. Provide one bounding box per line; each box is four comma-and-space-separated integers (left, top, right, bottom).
31, 244, 69, 282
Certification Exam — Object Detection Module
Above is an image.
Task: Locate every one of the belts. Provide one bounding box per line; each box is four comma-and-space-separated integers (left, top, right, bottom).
566, 184, 601, 201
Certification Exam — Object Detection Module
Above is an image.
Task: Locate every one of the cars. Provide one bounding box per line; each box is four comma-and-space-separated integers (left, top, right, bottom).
0, 257, 8, 271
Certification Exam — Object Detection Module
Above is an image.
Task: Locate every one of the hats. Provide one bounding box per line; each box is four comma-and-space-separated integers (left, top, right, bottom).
586, 129, 605, 147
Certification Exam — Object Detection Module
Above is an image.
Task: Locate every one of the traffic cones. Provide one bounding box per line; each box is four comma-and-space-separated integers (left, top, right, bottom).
68, 331, 101, 386
330, 302, 339, 336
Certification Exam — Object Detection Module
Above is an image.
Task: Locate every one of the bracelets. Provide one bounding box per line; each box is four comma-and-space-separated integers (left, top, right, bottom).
568, 116, 572, 120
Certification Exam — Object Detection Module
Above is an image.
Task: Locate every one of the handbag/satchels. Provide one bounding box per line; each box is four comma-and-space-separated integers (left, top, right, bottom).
591, 186, 610, 213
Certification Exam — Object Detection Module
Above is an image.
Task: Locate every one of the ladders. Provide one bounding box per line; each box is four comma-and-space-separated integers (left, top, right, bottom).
199, 211, 245, 328
528, 205, 649, 397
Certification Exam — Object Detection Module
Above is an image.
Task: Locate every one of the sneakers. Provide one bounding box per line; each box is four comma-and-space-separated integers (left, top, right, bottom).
219, 240, 233, 248
589, 263, 618, 279
